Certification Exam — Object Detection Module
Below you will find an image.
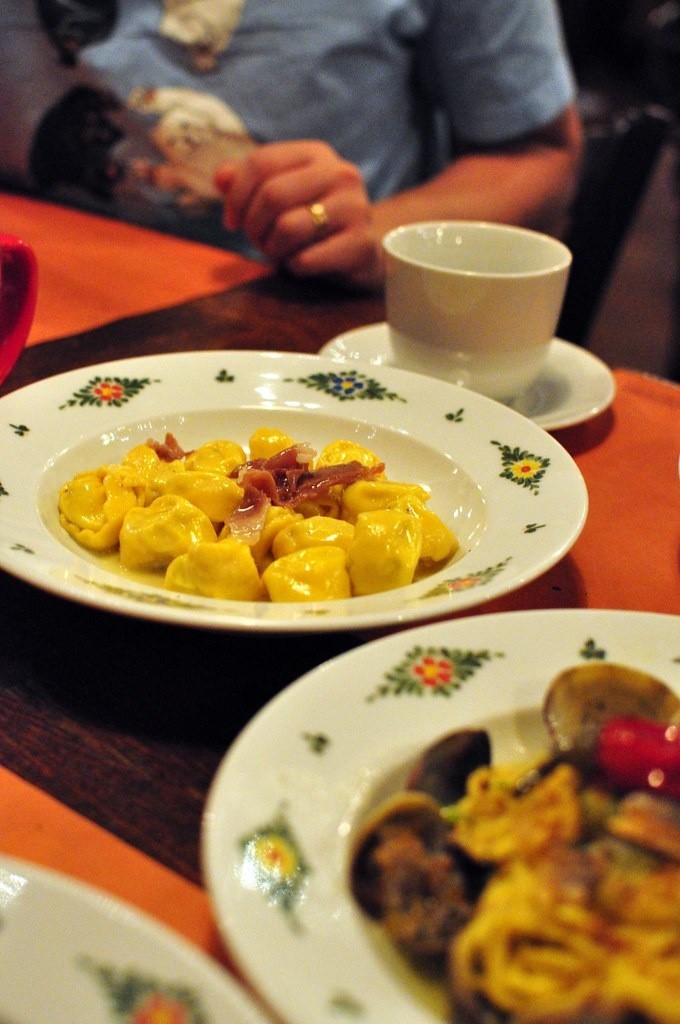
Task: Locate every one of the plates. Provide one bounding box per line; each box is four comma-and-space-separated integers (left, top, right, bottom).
0, 852, 273, 1024
318, 320, 618, 432
0, 349, 589, 633
199, 608, 680, 1024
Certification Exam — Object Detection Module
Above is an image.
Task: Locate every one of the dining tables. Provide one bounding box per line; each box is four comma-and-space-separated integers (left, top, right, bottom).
0, 191, 680, 1024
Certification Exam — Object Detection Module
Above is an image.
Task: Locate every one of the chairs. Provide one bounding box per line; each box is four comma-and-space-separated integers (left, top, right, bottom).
555, 105, 680, 383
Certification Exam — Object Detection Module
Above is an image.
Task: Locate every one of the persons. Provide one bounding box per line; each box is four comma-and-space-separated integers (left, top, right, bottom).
0, 0, 584, 297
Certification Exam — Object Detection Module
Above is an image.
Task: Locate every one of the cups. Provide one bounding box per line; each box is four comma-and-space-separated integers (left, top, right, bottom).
381, 220, 573, 402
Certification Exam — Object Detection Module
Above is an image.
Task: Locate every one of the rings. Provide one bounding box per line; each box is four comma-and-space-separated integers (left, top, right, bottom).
304, 201, 329, 243
